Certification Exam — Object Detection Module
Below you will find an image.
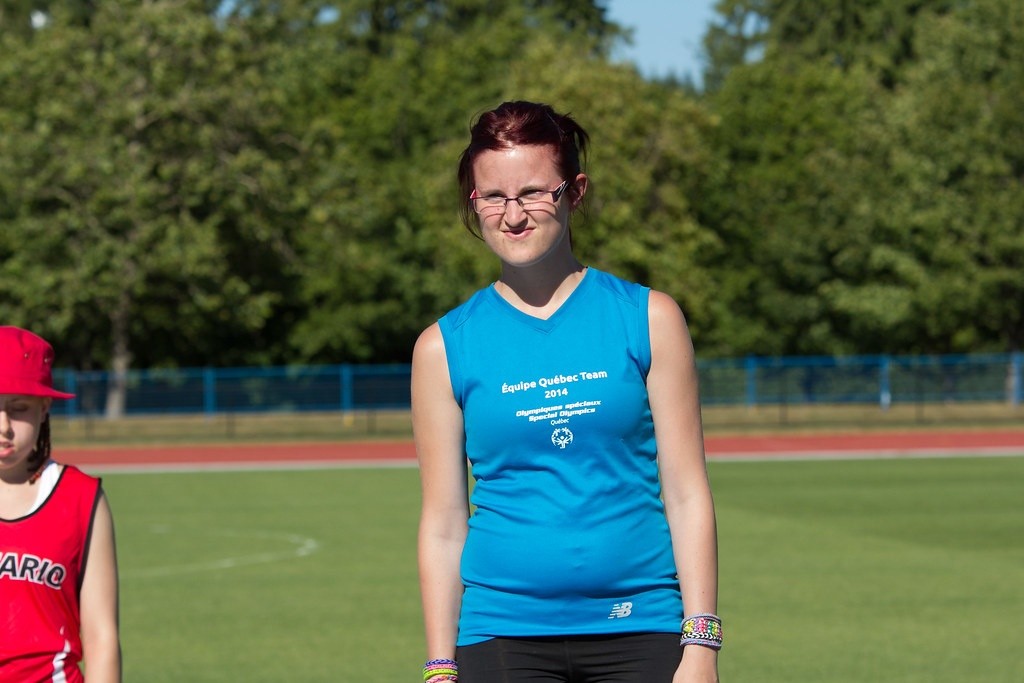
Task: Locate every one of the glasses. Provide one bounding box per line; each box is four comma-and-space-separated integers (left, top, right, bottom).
469, 181, 569, 215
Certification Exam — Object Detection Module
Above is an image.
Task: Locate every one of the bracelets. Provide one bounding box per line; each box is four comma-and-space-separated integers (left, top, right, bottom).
421, 660, 457, 682
681, 613, 721, 648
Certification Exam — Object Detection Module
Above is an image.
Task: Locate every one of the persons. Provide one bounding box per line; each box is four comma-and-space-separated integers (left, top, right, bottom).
409, 101, 719, 683
0, 327, 122, 683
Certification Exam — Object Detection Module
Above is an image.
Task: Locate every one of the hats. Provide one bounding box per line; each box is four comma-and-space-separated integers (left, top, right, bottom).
0, 325, 77, 400
